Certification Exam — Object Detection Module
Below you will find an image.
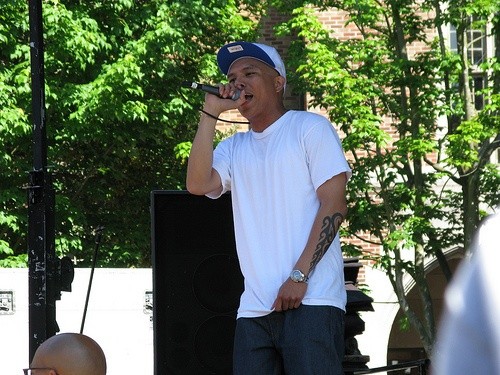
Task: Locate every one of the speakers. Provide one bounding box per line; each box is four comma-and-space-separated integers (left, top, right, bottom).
149, 190, 245, 375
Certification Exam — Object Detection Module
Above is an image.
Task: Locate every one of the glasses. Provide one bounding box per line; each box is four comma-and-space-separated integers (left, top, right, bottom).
23, 368, 57, 375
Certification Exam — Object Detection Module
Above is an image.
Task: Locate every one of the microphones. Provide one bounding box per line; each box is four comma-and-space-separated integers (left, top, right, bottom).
183, 81, 241, 101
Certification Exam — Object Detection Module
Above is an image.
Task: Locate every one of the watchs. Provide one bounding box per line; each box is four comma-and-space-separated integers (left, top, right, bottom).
290, 269, 309, 284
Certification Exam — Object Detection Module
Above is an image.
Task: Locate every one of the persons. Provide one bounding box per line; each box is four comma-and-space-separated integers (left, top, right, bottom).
186, 41, 353, 375
22, 333, 106, 375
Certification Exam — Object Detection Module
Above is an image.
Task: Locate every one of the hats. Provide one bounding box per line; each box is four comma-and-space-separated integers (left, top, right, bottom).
217, 41, 287, 98
